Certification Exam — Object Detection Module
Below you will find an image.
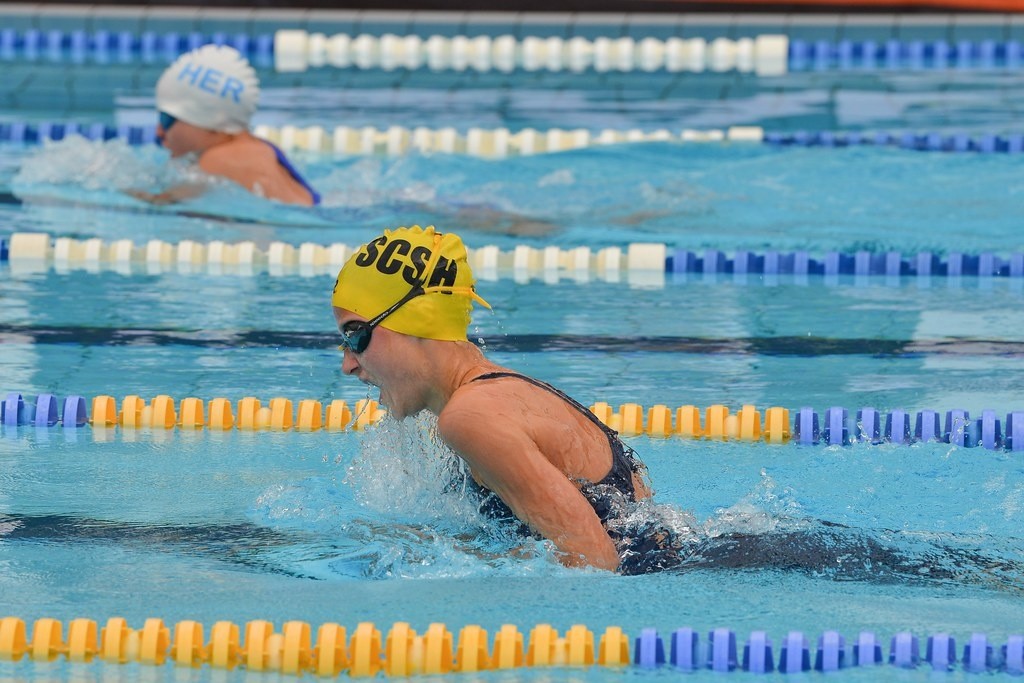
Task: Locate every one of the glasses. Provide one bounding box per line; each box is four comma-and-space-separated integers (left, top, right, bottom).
338, 280, 423, 353
160, 112, 177, 130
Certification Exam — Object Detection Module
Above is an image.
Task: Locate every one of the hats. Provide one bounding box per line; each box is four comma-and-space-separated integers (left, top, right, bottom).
154, 44, 260, 134
331, 225, 475, 342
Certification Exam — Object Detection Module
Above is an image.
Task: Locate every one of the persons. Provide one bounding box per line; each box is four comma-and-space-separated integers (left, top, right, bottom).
330, 224, 649, 573
152, 43, 324, 207
